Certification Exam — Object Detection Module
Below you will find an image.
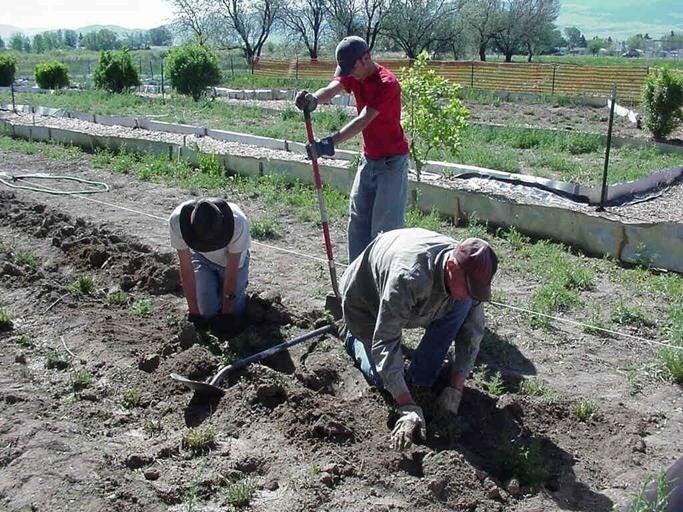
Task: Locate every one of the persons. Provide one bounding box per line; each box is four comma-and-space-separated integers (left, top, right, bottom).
295, 34, 409, 269
166, 196, 249, 323
333, 225, 499, 451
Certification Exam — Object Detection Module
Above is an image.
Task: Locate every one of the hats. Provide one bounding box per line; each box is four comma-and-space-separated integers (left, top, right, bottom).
334, 35, 369, 76
180, 198, 234, 253
454, 237, 497, 300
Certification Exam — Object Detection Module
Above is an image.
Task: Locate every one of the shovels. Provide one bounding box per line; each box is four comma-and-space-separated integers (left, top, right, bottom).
304, 104, 343, 321
170, 324, 332, 396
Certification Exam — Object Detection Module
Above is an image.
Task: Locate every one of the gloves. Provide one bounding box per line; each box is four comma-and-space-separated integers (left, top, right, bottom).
435, 388, 463, 422
295, 90, 317, 112
390, 406, 427, 450
304, 137, 335, 160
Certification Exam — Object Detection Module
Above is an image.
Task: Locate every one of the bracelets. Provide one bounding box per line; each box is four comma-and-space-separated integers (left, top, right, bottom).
223, 292, 235, 300
